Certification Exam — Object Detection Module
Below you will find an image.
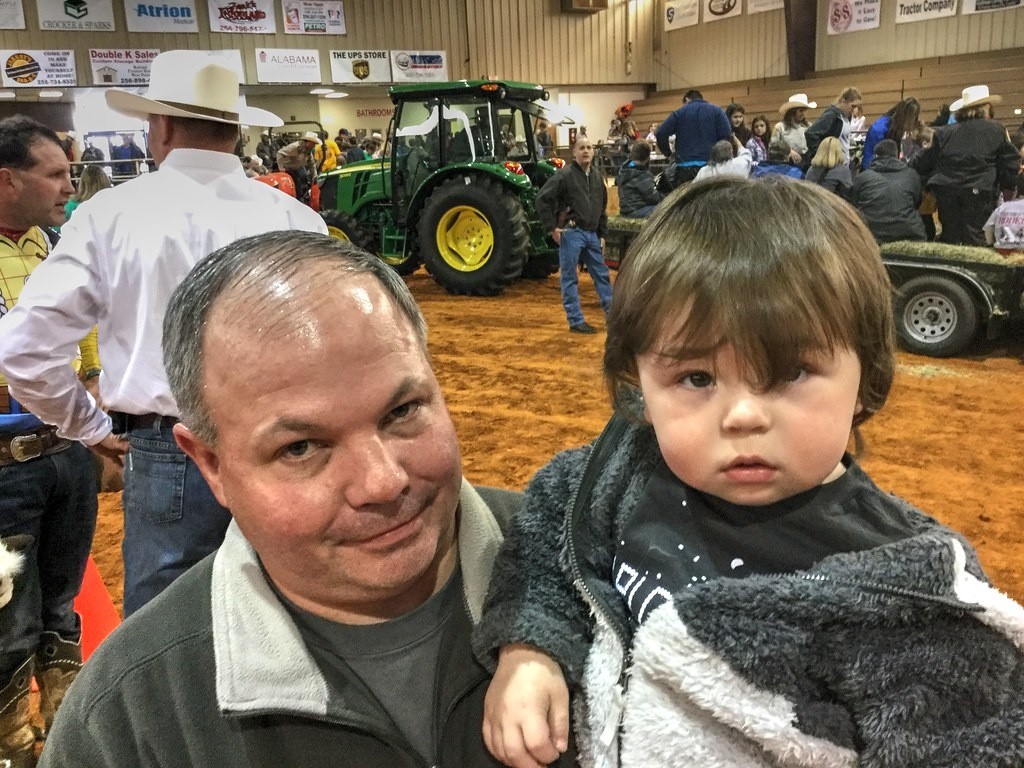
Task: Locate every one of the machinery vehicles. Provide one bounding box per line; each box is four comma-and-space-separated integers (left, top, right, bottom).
317, 80, 566, 297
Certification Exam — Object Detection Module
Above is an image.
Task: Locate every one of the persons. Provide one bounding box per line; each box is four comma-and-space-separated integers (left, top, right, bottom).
51, 165, 112, 232
82, 139, 104, 160
36, 232, 580, 768
60, 130, 76, 156
113, 134, 145, 173
471, 177, 1024, 768
537, 139, 612, 332
235, 122, 553, 202
0, 51, 329, 619
0, 120, 125, 768
615, 86, 1024, 244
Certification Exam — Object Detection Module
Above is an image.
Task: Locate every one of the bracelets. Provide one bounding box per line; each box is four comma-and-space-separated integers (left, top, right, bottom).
85, 369, 100, 379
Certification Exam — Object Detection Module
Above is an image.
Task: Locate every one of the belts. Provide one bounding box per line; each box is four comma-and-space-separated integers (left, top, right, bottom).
0, 423, 69, 466
131, 413, 183, 431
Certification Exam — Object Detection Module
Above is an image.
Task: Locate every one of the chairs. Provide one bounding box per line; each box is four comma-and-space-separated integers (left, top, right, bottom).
448, 107, 497, 155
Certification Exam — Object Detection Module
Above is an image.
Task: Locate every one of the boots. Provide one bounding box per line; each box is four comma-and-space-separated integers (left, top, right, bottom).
0, 651, 39, 768
40, 613, 85, 743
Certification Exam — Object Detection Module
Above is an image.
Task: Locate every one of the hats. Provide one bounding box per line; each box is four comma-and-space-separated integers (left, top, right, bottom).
251, 155, 263, 166
102, 50, 283, 129
259, 130, 269, 137
371, 133, 384, 142
339, 128, 350, 135
948, 84, 1003, 111
300, 131, 323, 145
85, 137, 95, 144
468, 106, 494, 119
66, 130, 78, 141
579, 124, 587, 129
778, 94, 818, 116
